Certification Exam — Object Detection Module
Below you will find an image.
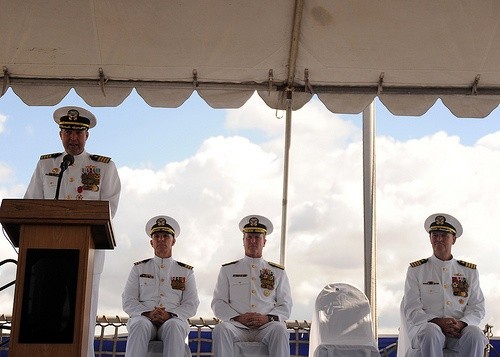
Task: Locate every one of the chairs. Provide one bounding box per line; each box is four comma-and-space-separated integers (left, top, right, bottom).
148, 283, 458, 357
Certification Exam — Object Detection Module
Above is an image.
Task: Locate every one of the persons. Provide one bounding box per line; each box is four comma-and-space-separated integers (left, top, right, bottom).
210, 215, 293, 357
122, 215, 200, 357
24, 106, 121, 357
403, 213, 489, 357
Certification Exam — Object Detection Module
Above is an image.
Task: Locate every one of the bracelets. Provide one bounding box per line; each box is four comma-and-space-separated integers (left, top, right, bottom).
266, 314, 275, 322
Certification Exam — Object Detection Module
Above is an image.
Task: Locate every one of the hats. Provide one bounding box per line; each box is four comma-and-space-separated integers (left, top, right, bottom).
424, 213, 463, 238
53, 106, 97, 132
239, 215, 274, 235
145, 215, 181, 239
